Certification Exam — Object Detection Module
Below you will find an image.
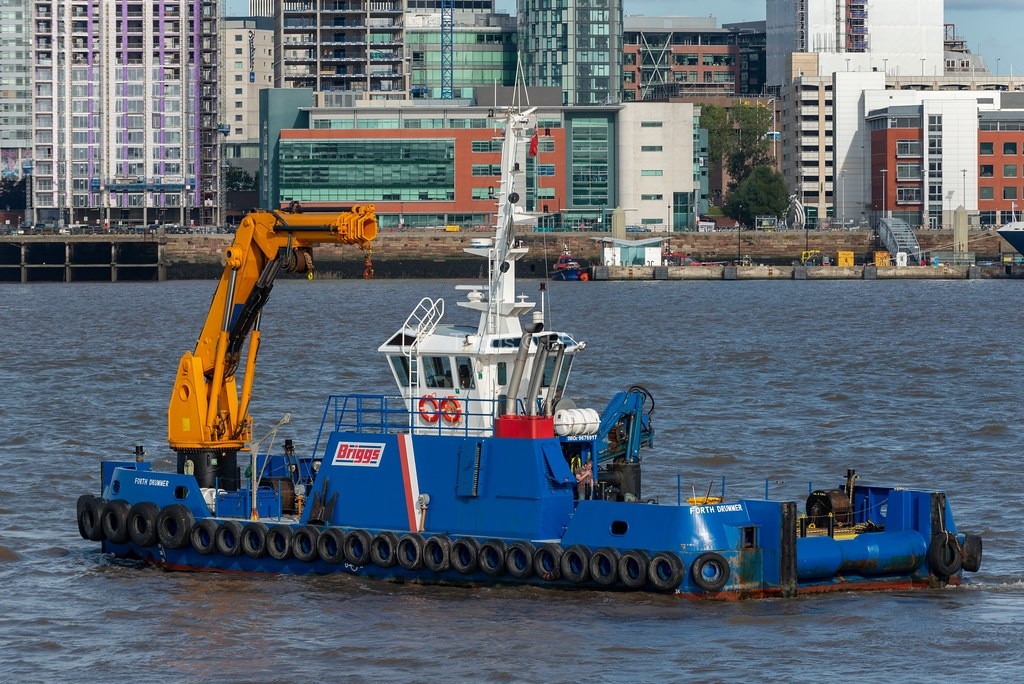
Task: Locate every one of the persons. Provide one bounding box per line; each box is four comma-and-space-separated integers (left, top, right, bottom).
575, 461, 594, 501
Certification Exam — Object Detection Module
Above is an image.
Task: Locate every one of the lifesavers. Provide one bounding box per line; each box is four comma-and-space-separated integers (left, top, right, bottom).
190, 519, 219, 555
317, 528, 346, 564
477, 539, 508, 575
216, 521, 244, 556
126, 502, 161, 547
423, 535, 453, 571
241, 524, 269, 558
369, 532, 398, 568
81, 497, 107, 541
396, 533, 426, 570
441, 396, 462, 423
420, 395, 440, 422
930, 532, 983, 578
342, 530, 372, 566
292, 526, 320, 561
505, 541, 733, 591
156, 504, 196, 549
450, 537, 479, 575
102, 499, 132, 544
77, 495, 94, 539
266, 526, 293, 559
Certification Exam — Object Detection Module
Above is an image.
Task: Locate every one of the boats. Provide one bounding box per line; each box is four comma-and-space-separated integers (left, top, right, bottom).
75, 48, 985, 602
544, 239, 594, 283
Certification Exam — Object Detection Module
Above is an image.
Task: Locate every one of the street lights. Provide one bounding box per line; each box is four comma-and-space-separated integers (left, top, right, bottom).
841, 169, 847, 230
961, 168, 968, 210
798, 170, 807, 230
878, 169, 889, 219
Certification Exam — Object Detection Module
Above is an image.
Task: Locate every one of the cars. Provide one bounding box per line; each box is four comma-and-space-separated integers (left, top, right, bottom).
0, 220, 238, 235
